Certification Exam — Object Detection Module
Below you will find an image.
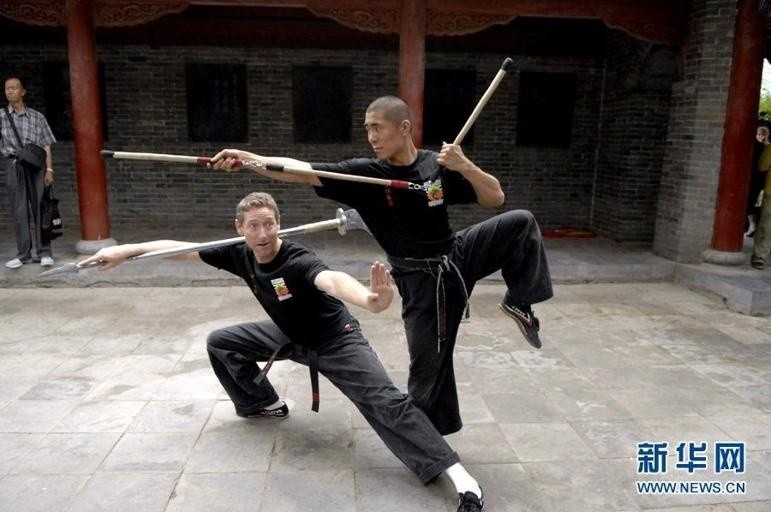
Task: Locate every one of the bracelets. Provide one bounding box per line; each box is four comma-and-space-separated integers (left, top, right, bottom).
45, 168, 55, 172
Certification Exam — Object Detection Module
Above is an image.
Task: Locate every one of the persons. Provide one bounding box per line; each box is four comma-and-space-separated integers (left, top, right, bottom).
744, 118, 771, 269
0, 74, 59, 268
78, 191, 484, 512
206, 96, 553, 435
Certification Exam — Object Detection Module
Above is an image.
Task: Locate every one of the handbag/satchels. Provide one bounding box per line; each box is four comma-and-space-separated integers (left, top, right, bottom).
18, 143, 46, 173
41, 198, 63, 240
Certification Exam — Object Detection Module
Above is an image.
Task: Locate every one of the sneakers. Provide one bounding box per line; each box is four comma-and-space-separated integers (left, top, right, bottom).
6, 254, 33, 269
40, 256, 54, 266
236, 401, 290, 419
498, 291, 541, 349
458, 485, 485, 512
751, 254, 764, 269
